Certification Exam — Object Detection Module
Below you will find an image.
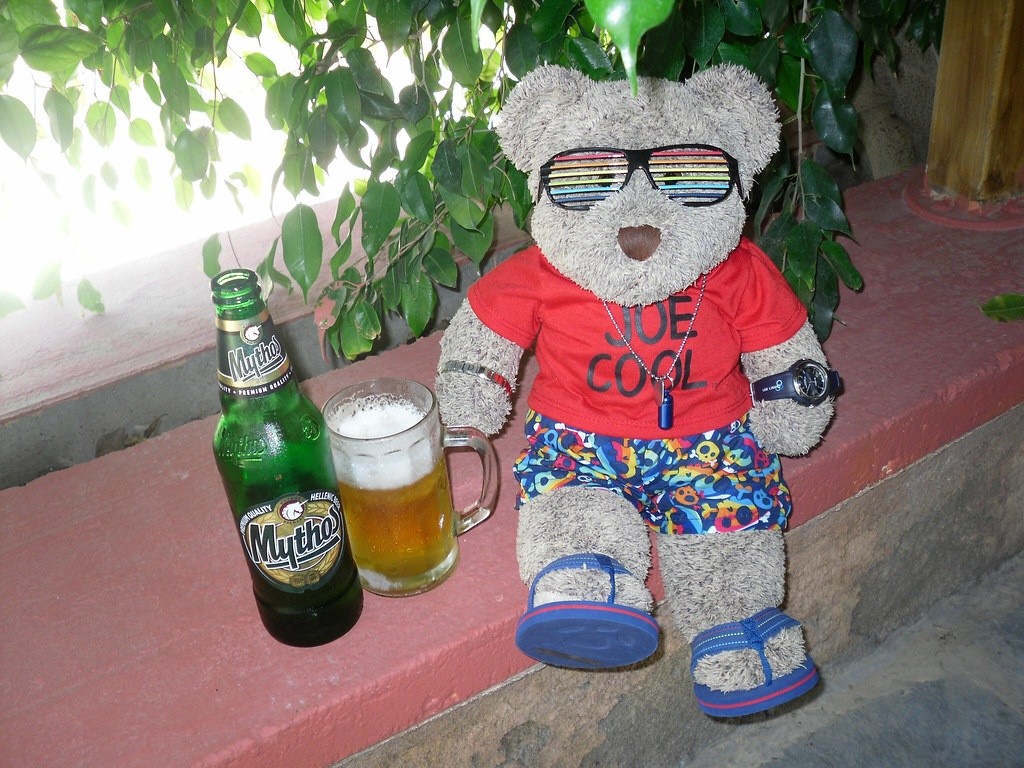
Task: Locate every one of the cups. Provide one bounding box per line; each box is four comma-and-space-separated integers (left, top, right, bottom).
321, 378, 498, 597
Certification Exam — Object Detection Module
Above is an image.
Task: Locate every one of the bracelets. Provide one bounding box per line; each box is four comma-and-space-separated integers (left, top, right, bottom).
435, 359, 512, 398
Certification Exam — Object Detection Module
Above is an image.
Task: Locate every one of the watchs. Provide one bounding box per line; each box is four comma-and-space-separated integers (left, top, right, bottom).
750, 358, 844, 408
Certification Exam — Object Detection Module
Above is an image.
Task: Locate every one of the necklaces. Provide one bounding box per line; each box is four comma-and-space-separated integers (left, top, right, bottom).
602, 272, 705, 428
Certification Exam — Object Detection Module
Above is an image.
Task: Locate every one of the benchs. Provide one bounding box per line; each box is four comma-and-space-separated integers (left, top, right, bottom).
0, 164, 1023, 768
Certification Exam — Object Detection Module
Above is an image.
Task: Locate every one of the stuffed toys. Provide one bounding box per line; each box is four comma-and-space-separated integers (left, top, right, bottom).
434, 64, 836, 720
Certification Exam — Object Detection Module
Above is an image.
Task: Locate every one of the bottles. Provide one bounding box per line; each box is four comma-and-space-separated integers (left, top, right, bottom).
211, 270, 363, 647
